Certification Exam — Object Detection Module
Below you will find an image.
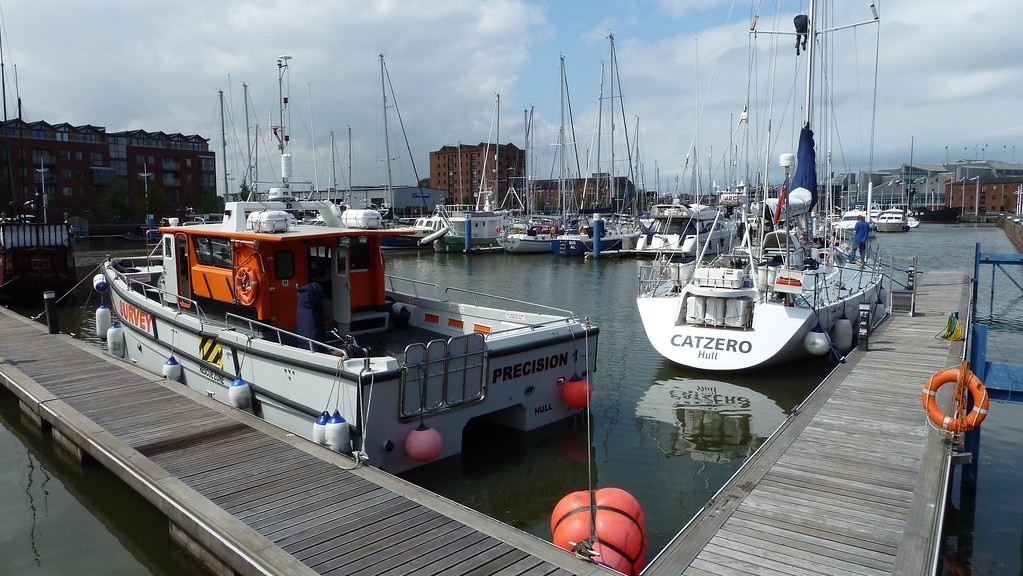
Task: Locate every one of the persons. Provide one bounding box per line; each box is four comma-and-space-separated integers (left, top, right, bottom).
793, 14, 810, 56
849, 215, 868, 265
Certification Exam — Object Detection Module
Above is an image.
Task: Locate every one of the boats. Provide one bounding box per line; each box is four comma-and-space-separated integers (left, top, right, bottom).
909, 189, 965, 224
634, 356, 838, 462
92, 55, 601, 476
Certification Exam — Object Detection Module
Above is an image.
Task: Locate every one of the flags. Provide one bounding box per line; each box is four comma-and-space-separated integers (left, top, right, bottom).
772, 177, 788, 225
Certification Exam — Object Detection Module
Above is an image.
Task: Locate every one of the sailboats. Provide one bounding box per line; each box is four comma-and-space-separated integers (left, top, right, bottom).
218, 1, 922, 370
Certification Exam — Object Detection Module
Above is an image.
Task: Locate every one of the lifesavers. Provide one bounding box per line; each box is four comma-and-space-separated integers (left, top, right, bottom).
495, 226, 500, 233
922, 367, 989, 433
776, 278, 801, 286
234, 266, 258, 306
